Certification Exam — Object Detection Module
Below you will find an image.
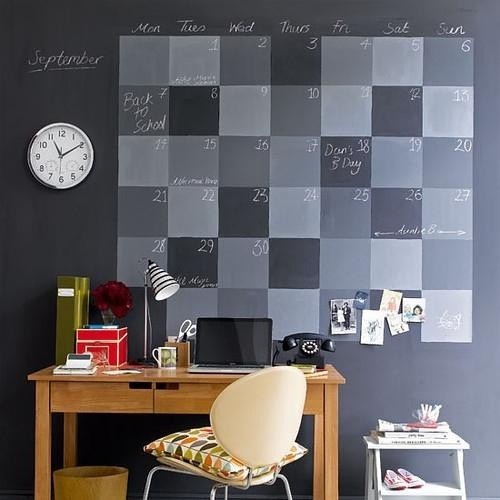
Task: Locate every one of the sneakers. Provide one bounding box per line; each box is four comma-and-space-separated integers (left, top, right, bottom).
384, 468, 425, 489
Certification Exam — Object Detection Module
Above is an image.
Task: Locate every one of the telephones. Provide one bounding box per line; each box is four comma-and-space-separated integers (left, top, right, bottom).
282, 332, 335, 369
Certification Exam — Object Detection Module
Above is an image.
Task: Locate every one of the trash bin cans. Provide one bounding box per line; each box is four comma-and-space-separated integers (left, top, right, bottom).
53, 466, 129, 500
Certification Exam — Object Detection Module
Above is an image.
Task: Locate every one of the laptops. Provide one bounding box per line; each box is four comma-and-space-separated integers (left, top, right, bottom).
187, 316, 272, 374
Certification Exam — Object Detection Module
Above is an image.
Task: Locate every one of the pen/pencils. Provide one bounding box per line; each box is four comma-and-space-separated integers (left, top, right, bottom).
417, 403, 442, 420
182, 333, 187, 342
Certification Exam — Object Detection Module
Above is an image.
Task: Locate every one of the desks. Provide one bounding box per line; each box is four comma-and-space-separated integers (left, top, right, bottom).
362, 435, 471, 500
26, 360, 349, 500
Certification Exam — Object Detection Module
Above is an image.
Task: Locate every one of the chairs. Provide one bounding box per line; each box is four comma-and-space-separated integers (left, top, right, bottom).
140, 365, 309, 500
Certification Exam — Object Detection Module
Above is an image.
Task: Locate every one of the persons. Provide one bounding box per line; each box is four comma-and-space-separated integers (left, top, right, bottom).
412, 305, 423, 321
334, 301, 352, 330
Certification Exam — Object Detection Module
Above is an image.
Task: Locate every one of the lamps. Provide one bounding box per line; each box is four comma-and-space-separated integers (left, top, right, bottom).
132, 259, 180, 367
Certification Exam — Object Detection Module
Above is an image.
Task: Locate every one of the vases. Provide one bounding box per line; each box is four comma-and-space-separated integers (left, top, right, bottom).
100, 309, 115, 326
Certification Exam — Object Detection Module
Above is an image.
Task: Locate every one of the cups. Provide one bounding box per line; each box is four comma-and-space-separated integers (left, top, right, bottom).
152, 346, 178, 370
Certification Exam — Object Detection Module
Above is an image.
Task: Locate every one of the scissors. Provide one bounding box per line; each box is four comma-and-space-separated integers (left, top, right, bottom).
177, 320, 197, 343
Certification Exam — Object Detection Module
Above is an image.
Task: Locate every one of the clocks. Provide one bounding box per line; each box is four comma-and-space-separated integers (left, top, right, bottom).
25, 122, 96, 192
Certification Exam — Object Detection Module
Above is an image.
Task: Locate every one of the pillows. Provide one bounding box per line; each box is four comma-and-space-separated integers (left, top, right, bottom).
142, 423, 307, 482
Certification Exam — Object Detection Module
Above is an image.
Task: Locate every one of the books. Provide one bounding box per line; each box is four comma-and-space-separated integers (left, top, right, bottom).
369, 432, 460, 446
291, 364, 328, 379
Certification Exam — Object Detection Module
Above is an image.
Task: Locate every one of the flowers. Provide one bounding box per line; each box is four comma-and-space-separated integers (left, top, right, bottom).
90, 280, 135, 318
